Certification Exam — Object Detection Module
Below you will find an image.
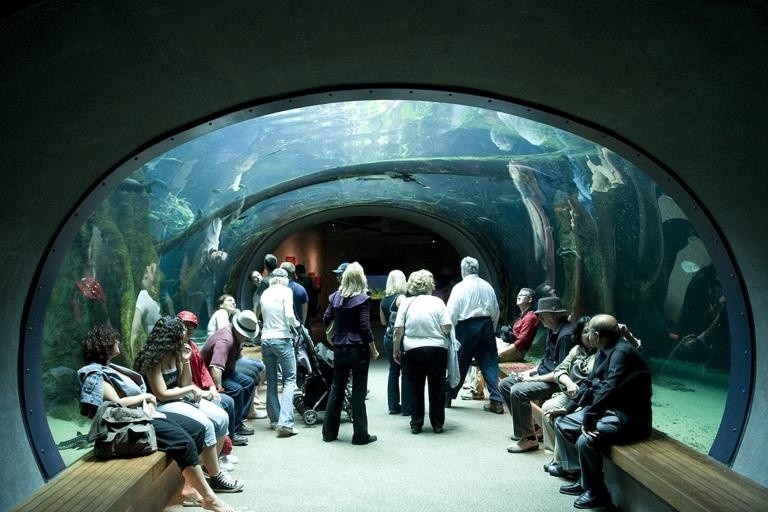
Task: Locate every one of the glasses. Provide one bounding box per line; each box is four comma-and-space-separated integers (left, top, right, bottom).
517, 292, 531, 298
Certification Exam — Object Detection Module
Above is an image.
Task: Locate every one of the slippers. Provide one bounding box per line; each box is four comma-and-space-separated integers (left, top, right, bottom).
180, 494, 206, 507
232, 509, 254, 512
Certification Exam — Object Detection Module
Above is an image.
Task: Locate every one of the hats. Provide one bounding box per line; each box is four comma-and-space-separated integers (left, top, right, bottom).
332, 263, 349, 274
233, 310, 263, 338
272, 269, 288, 276
534, 297, 569, 314
179, 311, 204, 328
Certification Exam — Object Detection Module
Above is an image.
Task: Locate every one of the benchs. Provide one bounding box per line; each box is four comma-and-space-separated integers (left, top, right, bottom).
500, 360, 768, 512
6, 346, 263, 512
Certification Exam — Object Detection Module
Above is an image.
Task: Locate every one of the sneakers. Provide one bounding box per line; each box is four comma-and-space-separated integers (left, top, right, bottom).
510, 428, 543, 439
219, 463, 234, 471
507, 438, 541, 452
252, 400, 267, 409
212, 476, 245, 492
246, 409, 267, 420
223, 455, 240, 463
278, 426, 300, 435
270, 423, 277, 430
462, 389, 484, 401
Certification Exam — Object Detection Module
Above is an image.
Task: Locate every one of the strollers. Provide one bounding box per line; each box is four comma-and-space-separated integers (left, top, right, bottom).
293, 326, 354, 425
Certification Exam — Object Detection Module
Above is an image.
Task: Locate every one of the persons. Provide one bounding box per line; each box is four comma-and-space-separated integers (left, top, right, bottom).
133, 315, 244, 493
256, 253, 278, 321
332, 262, 351, 284
460, 288, 541, 400
258, 268, 299, 437
229, 309, 266, 410
295, 264, 315, 335
130, 263, 175, 359
536, 283, 558, 299
200, 309, 259, 446
380, 270, 415, 417
497, 296, 576, 453
248, 270, 263, 313
322, 262, 380, 446
559, 314, 653, 509
75, 324, 235, 512
445, 256, 504, 414
542, 315, 643, 482
392, 269, 452, 435
194, 218, 230, 276
280, 262, 309, 327
206, 294, 267, 419
178, 310, 238, 472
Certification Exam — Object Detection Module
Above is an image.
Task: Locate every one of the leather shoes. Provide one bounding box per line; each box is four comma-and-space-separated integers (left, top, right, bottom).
389, 406, 411, 416
445, 393, 452, 407
557, 479, 584, 495
232, 434, 248, 445
238, 423, 257, 435
484, 400, 504, 413
411, 426, 420, 435
573, 490, 609, 508
367, 434, 379, 441
434, 425, 443, 433
543, 457, 569, 478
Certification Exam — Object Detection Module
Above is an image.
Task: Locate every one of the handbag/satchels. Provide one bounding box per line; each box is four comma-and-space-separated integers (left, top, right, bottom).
325, 292, 339, 347
74, 368, 158, 460
559, 363, 591, 397
387, 293, 401, 333
